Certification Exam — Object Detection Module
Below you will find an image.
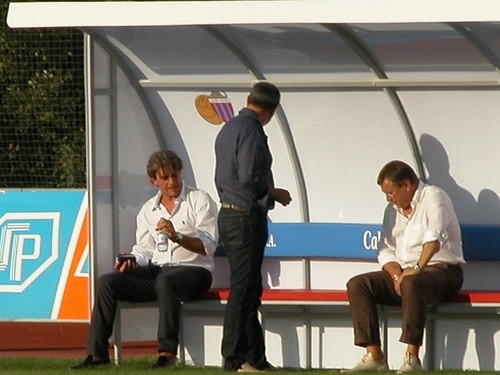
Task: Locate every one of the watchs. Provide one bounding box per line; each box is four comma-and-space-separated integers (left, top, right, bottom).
412, 263, 422, 271
175, 232, 183, 244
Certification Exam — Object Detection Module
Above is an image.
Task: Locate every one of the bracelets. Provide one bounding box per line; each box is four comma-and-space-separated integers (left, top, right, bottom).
392, 273, 402, 281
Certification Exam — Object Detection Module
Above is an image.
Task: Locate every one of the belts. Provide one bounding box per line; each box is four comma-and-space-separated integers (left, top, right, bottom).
222, 203, 244, 210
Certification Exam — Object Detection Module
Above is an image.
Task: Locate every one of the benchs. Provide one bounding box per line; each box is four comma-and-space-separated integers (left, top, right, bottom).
111, 223, 500, 369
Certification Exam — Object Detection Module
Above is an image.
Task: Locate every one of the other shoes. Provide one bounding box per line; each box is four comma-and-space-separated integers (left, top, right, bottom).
152, 355, 175, 369
396, 353, 421, 375
223, 357, 273, 373
341, 353, 390, 374
70, 353, 109, 368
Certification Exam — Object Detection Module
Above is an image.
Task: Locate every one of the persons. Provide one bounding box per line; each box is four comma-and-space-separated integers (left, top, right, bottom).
340, 160, 466, 375
70, 150, 220, 372
214, 82, 293, 374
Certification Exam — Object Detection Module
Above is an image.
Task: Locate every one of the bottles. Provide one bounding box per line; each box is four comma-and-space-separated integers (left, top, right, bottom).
156, 231, 168, 252
